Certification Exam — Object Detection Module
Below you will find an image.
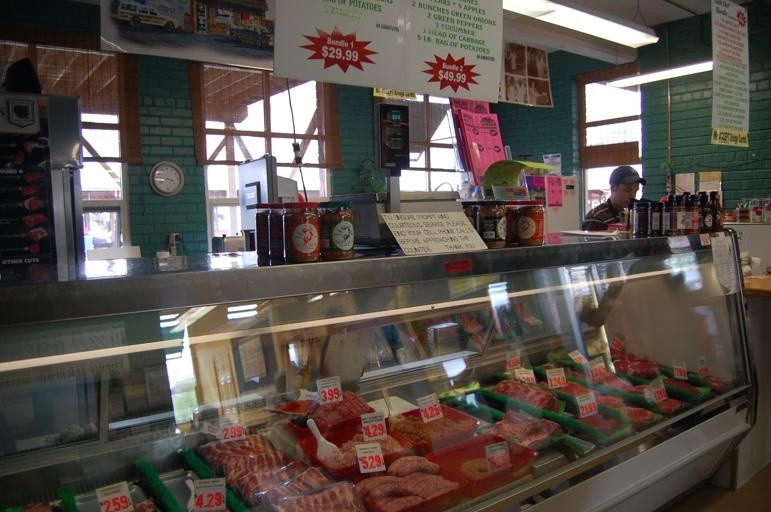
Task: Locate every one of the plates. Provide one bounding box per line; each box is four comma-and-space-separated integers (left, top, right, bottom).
385, 404, 482, 456
299, 423, 414, 479
425, 432, 538, 499
275, 389, 377, 439
352, 468, 465, 512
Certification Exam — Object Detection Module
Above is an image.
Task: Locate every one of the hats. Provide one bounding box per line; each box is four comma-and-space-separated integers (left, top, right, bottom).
609, 165, 646, 187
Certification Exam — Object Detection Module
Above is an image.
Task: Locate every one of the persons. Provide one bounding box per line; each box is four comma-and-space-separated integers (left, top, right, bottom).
581, 165, 647, 232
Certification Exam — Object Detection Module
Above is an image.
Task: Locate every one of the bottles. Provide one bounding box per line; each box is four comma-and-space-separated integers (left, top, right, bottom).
476, 200, 507, 249
320, 201, 355, 260
505, 199, 519, 243
516, 200, 545, 247
460, 200, 477, 230
268, 202, 285, 260
648, 201, 662, 237
255, 202, 270, 256
284, 202, 320, 263
634, 202, 649, 239
661, 191, 722, 236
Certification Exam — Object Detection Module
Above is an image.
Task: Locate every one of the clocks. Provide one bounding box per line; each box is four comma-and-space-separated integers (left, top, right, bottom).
150, 161, 185, 196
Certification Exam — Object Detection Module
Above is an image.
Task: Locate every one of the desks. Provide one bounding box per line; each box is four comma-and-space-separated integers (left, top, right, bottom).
711, 273, 770, 492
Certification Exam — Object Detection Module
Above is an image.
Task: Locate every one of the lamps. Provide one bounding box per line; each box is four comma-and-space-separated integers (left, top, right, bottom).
503, 0, 659, 49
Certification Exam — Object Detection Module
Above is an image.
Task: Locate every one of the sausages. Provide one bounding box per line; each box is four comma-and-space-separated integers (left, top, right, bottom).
358, 476, 422, 511
387, 455, 427, 477
398, 461, 441, 479
402, 474, 459, 503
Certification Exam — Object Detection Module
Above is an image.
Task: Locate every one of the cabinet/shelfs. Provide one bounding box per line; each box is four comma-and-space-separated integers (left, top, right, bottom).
2, 226, 756, 512
